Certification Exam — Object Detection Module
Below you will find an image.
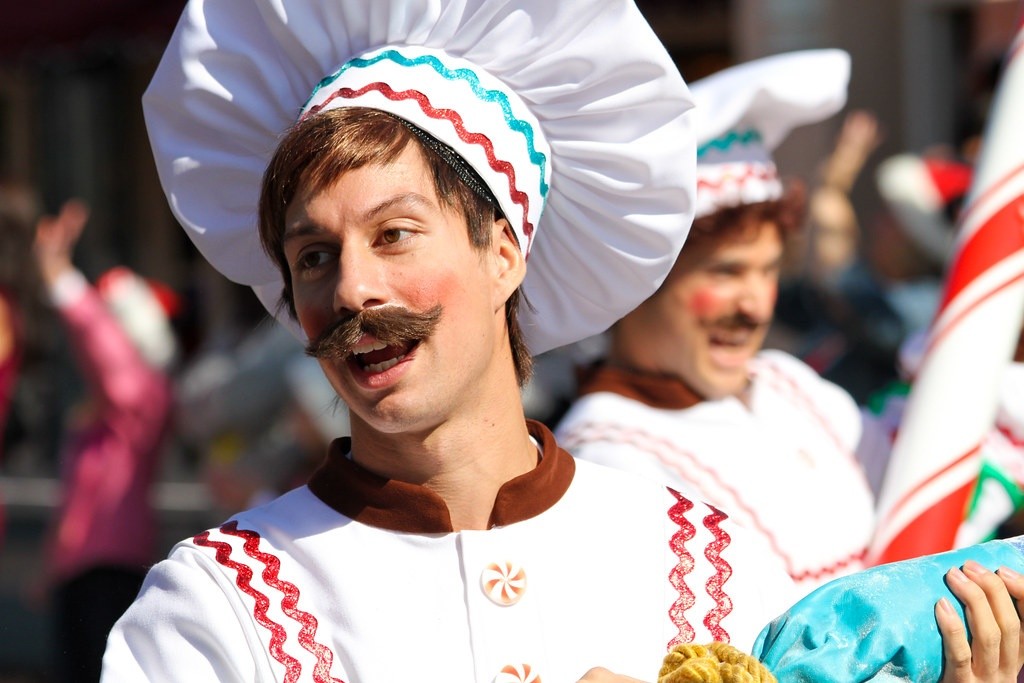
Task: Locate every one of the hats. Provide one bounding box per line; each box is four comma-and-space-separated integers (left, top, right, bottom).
142, 1, 697, 359
680, 50, 850, 221
103, 266, 207, 381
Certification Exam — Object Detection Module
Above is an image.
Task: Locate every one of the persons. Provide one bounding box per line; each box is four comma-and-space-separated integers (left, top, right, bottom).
100, 50, 1024, 683
0, 167, 353, 683
554, 136, 1024, 589
